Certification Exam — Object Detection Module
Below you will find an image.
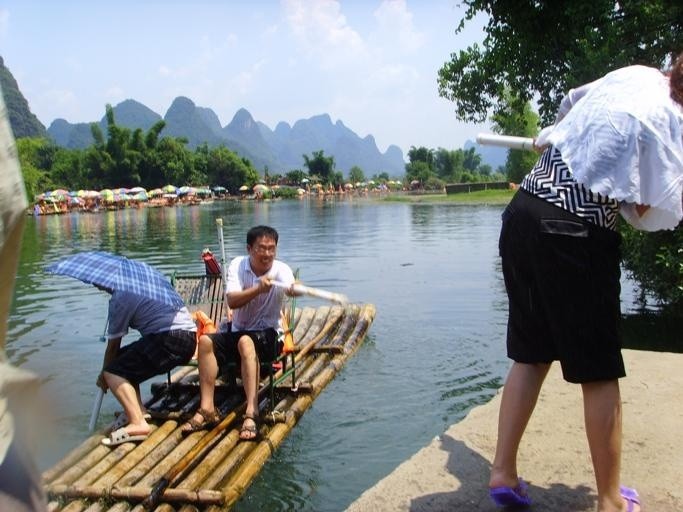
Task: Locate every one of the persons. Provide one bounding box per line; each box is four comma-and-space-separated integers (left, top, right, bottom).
0, 86, 47, 512
93, 282, 199, 446
182, 225, 302, 441
489, 53, 683, 512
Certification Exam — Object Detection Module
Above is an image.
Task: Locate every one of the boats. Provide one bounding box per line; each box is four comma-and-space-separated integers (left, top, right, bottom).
26, 192, 281, 215
297, 188, 390, 200
37, 301, 377, 511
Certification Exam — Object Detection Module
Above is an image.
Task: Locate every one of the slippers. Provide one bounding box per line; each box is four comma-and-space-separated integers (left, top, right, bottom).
103, 412, 152, 445
618, 486, 642, 511
491, 476, 531, 507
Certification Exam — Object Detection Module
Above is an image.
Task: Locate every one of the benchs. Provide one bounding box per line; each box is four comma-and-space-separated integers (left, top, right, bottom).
167, 269, 301, 418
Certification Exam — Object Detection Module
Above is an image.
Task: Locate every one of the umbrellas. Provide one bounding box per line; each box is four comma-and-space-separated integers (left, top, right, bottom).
44, 250, 185, 433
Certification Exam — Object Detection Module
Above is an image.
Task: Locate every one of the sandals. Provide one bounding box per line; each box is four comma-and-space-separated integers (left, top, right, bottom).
181, 407, 222, 434
239, 415, 260, 441
